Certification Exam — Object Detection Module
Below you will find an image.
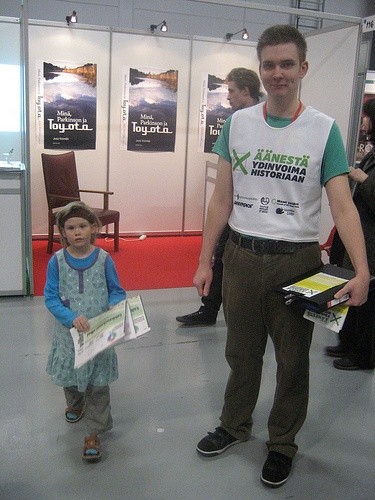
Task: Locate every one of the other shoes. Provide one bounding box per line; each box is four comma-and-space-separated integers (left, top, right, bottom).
333, 358, 360, 370
260, 450, 292, 486
195, 427, 243, 457
326, 346, 347, 357
176, 306, 216, 324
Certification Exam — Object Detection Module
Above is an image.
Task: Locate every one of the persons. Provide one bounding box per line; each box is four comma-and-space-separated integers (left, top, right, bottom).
325, 98, 375, 370
196, 26, 370, 488
44, 202, 126, 462
179, 68, 268, 325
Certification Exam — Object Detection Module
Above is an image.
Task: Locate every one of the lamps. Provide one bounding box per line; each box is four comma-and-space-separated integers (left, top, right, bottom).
150, 21, 167, 33
65, 10, 77, 25
225, 29, 248, 41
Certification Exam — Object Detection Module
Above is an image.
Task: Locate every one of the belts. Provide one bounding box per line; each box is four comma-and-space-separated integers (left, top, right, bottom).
227, 230, 316, 255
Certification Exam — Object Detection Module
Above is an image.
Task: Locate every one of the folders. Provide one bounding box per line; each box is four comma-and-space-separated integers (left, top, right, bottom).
273, 263, 375, 312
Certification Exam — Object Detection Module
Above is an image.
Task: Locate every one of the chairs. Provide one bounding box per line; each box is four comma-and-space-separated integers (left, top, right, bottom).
41, 151, 120, 253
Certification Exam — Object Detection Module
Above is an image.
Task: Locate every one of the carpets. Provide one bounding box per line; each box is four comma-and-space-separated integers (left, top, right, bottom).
31, 236, 203, 296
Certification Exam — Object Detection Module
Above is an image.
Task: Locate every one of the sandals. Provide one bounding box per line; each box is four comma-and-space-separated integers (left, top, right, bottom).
82, 432, 102, 460
64, 402, 85, 423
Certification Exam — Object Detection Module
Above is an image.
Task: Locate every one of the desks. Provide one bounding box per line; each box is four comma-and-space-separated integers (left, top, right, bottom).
0, 160, 27, 297
